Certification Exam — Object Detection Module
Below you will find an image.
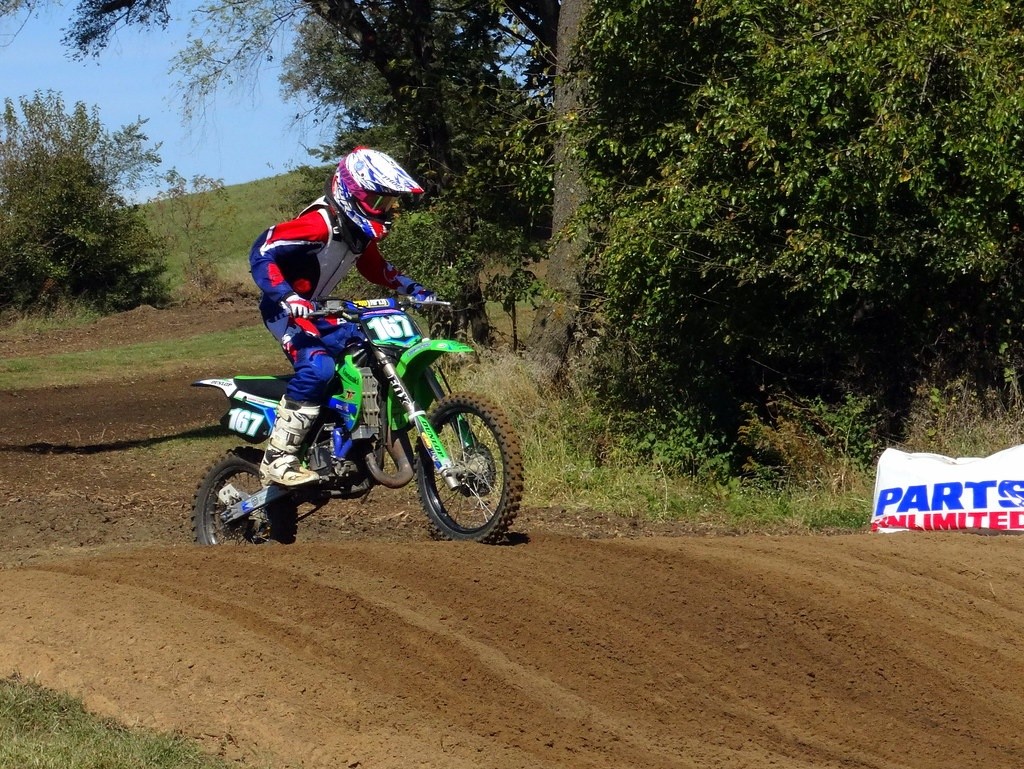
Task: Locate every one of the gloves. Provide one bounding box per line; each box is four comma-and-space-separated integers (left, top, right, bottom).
411, 287, 434, 305
287, 294, 315, 318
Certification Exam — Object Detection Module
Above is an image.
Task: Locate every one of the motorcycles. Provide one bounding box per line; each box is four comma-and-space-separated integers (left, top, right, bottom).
190, 295, 525, 545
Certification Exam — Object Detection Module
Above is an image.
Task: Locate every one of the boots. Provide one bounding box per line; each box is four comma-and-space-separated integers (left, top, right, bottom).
259, 393, 321, 487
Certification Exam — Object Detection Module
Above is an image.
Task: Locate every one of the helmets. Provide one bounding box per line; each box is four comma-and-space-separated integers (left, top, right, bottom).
332, 150, 423, 241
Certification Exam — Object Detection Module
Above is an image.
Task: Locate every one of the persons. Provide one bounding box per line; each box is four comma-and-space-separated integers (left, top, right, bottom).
249, 146, 437, 486
